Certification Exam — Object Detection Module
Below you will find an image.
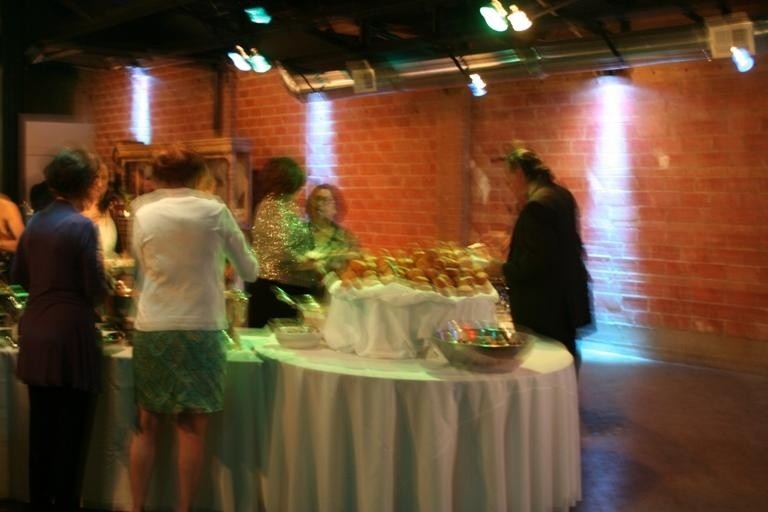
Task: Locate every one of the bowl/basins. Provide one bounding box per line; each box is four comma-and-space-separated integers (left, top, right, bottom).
273, 324, 324, 350
430, 326, 538, 375
266, 318, 300, 332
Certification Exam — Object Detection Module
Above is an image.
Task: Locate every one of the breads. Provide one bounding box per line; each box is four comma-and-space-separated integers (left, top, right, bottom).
340, 239, 492, 296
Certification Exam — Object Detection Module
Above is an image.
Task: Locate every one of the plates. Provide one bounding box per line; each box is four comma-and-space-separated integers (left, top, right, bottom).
106, 332, 125, 343
306, 250, 361, 261
101, 257, 136, 271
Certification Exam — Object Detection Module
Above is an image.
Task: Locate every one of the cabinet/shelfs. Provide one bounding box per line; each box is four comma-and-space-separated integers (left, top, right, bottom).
113, 136, 252, 257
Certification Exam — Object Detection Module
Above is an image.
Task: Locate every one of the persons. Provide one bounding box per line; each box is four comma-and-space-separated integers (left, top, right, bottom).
475, 147, 591, 361
0, 158, 363, 329
111, 154, 260, 512
7, 146, 109, 512
